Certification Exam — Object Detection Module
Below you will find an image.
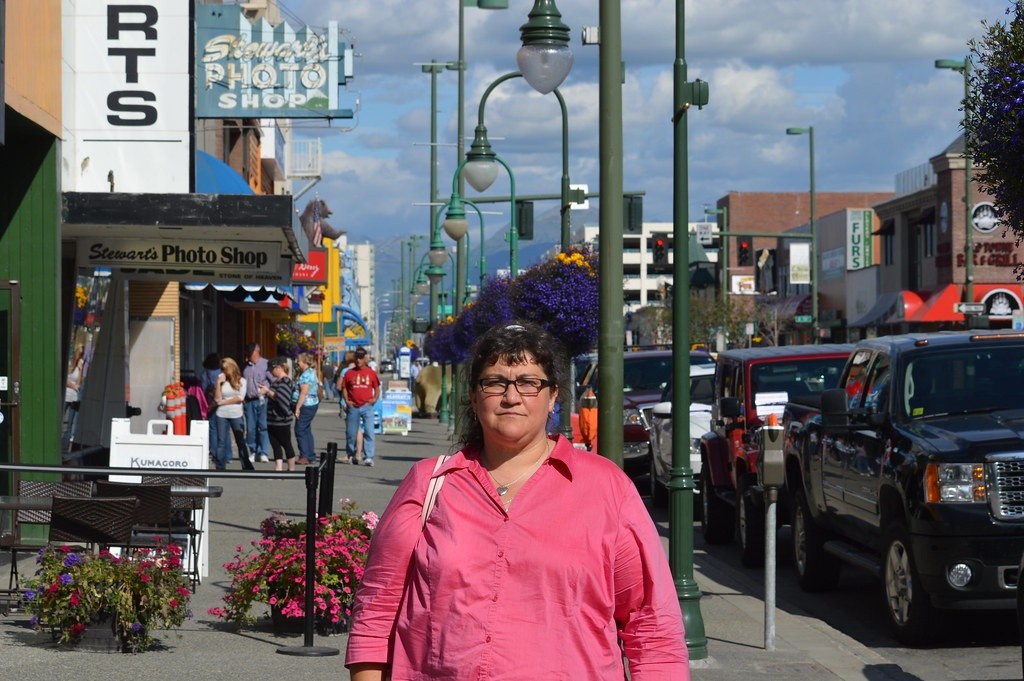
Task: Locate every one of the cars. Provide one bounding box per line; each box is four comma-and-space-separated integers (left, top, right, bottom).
648, 364, 715, 520
572, 345, 715, 494
380, 360, 395, 374
698, 344, 877, 568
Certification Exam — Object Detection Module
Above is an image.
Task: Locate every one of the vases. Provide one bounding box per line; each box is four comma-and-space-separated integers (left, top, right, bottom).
267, 578, 305, 628
114, 596, 146, 647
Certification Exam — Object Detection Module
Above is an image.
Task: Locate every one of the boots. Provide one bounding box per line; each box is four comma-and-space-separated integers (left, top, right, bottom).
288, 456, 296, 471
275, 459, 283, 472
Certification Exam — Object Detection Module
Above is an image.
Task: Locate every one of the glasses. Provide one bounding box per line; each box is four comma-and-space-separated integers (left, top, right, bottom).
476, 378, 553, 394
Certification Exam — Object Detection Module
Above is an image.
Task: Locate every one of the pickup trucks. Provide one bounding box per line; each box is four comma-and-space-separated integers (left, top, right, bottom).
783, 328, 1024, 643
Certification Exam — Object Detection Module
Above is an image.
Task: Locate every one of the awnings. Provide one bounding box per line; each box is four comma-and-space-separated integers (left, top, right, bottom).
848, 291, 900, 328
766, 292, 819, 330
885, 290, 925, 322
185, 149, 295, 302
963, 284, 1024, 321
908, 284, 966, 322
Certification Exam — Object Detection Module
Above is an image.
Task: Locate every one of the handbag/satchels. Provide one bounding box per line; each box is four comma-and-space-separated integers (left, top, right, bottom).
317, 385, 323, 402
204, 383, 218, 417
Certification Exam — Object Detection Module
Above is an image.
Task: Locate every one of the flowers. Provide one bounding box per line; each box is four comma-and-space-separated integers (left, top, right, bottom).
208, 497, 381, 636
424, 238, 600, 366
275, 324, 316, 356
402, 339, 421, 362
9, 536, 193, 655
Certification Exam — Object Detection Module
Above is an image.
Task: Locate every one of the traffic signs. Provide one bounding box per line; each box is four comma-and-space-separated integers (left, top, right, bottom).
696, 223, 712, 244
955, 303, 986, 313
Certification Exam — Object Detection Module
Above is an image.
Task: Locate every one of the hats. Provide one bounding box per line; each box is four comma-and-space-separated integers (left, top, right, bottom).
355, 348, 367, 358
266, 357, 284, 371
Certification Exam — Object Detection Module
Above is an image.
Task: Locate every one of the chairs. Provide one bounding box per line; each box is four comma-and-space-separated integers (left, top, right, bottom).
97, 479, 173, 557
926, 362, 964, 400
908, 366, 930, 417
132, 476, 207, 594
759, 371, 785, 392
47, 496, 138, 558
4, 480, 94, 616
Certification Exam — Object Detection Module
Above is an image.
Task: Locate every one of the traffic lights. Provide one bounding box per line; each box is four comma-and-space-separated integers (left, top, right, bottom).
736, 236, 752, 266
651, 234, 668, 267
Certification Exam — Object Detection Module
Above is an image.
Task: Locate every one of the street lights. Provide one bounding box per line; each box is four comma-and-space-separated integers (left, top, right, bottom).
934, 57, 974, 331
428, 199, 486, 290
459, 73, 574, 444
445, 153, 516, 280
786, 126, 820, 345
516, 0, 710, 665
704, 207, 727, 350
407, 223, 470, 441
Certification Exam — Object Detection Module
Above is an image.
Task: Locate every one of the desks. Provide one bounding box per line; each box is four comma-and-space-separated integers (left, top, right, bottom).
169, 485, 223, 498
0, 495, 54, 510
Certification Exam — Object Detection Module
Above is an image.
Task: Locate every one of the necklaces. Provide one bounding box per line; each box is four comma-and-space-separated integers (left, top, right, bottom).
480, 441, 549, 506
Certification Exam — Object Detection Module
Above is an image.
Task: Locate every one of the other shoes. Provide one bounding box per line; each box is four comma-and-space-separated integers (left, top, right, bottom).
337, 455, 352, 464
259, 454, 268, 462
364, 458, 374, 466
355, 454, 363, 464
242, 462, 254, 471
295, 456, 309, 465
216, 464, 226, 472
249, 454, 256, 463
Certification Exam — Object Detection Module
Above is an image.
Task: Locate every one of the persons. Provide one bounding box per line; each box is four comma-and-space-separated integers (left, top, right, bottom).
323, 346, 381, 467
344, 317, 689, 681
63, 343, 85, 452
239, 343, 320, 471
201, 353, 235, 464
214, 358, 256, 472
411, 360, 422, 398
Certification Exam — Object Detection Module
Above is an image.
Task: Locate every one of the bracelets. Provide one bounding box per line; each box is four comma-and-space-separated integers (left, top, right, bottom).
266, 390, 269, 394
